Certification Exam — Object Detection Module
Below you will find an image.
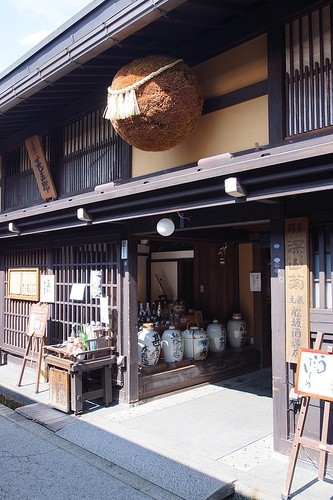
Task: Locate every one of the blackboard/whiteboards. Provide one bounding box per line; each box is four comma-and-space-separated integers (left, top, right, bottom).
295, 348, 333, 402
26, 304, 50, 338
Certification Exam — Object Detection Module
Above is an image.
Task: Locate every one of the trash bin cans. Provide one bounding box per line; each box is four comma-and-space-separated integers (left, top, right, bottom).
0, 349, 6, 366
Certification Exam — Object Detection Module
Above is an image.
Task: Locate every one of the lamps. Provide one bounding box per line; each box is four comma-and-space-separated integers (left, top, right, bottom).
156, 213, 185, 237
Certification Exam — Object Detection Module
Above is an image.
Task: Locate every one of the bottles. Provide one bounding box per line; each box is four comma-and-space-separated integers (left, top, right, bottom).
138, 301, 169, 321
64, 319, 102, 362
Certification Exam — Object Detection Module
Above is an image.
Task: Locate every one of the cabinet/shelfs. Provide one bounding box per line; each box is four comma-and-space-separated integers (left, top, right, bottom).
43, 326, 116, 415
